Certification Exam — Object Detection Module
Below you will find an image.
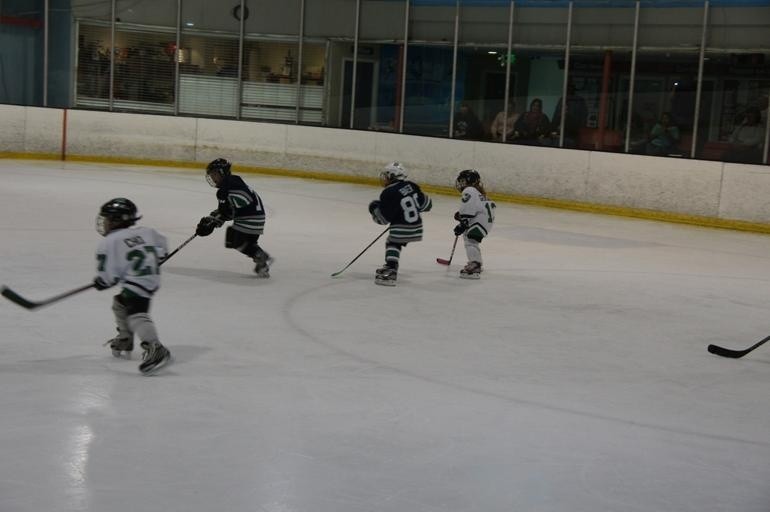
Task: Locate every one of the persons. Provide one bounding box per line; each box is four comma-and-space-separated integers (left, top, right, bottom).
552, 79, 589, 137
450, 104, 473, 139
492, 100, 520, 141
514, 98, 550, 139
730, 108, 764, 148
610, 99, 643, 140
647, 112, 680, 158
369, 162, 433, 286
454, 170, 496, 279
197, 158, 275, 280
94, 198, 170, 373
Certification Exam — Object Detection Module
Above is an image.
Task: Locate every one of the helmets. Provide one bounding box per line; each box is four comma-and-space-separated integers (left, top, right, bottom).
380, 161, 408, 187
97, 198, 137, 236
206, 158, 231, 187
114, 48, 120, 57
456, 170, 480, 193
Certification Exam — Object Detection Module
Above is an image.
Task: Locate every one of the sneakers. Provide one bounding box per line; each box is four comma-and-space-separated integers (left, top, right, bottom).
111, 337, 133, 350
140, 341, 171, 373
375, 271, 397, 280
461, 263, 473, 274
376, 264, 390, 274
465, 261, 481, 273
261, 252, 268, 260
255, 263, 269, 274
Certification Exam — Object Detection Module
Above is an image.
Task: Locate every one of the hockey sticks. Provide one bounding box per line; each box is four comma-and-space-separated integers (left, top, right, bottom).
709, 337, 769, 360
330, 227, 391, 276
437, 234, 459, 265
1, 279, 101, 311
158, 214, 222, 267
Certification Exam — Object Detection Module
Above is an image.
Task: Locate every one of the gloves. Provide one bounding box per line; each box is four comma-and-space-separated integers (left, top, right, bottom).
454, 222, 468, 236
200, 216, 213, 225
196, 220, 217, 237
369, 201, 381, 214
455, 211, 460, 221
93, 277, 108, 291
